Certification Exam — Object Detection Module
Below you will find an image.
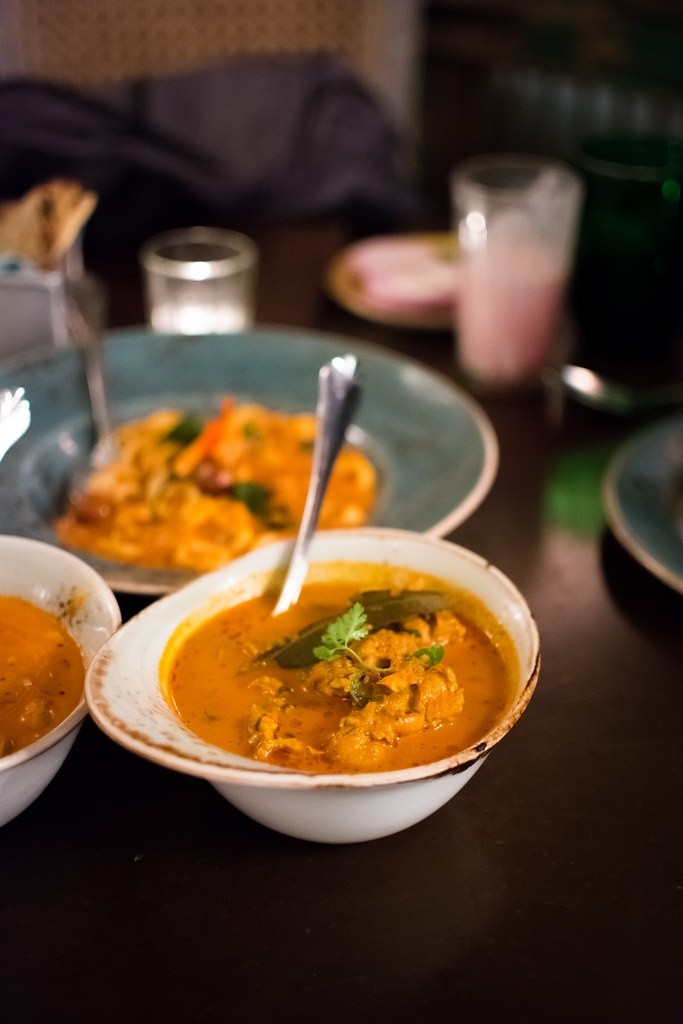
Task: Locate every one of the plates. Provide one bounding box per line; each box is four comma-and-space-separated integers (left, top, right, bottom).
602, 417, 683, 594
327, 233, 460, 329
0, 321, 499, 595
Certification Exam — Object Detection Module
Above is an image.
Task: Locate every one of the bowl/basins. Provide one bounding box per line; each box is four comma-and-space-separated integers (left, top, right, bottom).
83, 527, 542, 843
0, 534, 122, 827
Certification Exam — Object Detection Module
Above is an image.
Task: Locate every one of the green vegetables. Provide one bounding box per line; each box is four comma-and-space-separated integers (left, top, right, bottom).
160, 411, 289, 528
314, 603, 446, 707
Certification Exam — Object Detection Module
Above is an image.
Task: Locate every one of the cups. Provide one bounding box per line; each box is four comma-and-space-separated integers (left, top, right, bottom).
452, 156, 583, 396
143, 228, 256, 335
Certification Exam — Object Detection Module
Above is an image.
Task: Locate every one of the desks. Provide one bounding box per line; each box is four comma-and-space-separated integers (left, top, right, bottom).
0, 229, 683, 1024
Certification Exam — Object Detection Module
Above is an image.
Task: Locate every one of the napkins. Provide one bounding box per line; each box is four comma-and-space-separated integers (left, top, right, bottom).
0, 180, 97, 272
